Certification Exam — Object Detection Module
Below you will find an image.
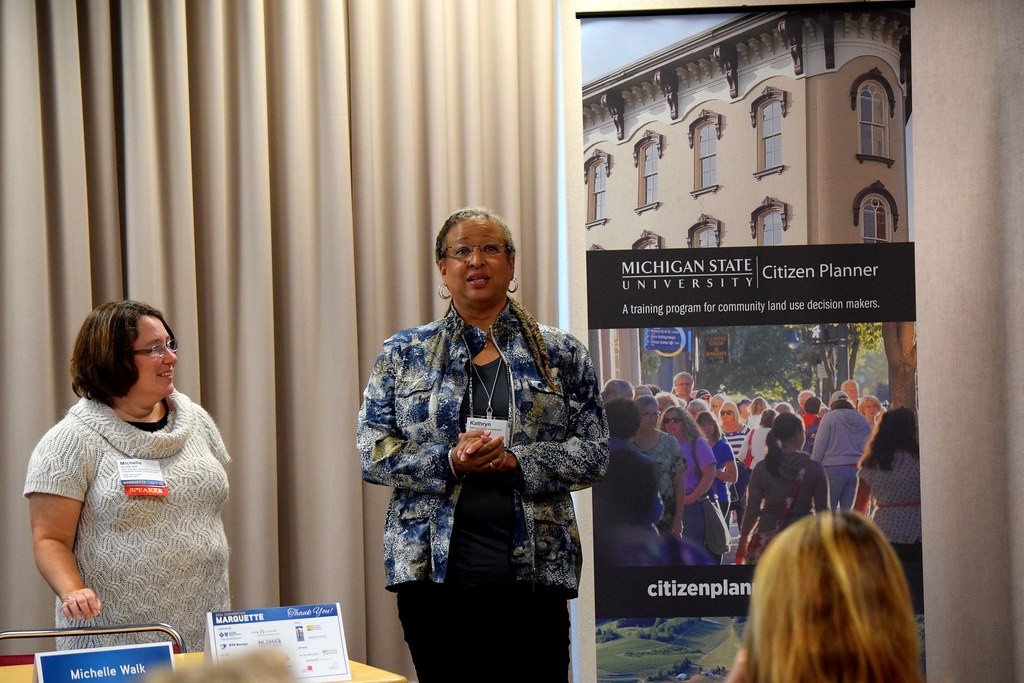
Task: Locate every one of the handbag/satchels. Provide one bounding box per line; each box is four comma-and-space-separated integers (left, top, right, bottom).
702, 500, 732, 555
730, 483, 739, 502
743, 429, 755, 469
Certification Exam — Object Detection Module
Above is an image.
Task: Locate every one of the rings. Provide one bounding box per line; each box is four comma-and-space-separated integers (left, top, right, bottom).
489, 461, 495, 469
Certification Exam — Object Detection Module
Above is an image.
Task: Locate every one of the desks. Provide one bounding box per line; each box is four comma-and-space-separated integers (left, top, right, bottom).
0, 651, 408, 683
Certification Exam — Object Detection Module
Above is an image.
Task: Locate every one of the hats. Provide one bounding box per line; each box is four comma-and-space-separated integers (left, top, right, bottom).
829, 391, 850, 406
740, 398, 753, 406
696, 389, 711, 399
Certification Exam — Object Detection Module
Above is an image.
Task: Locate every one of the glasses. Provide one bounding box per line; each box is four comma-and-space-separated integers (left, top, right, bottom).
720, 410, 732, 415
604, 389, 616, 395
663, 418, 681, 424
447, 243, 506, 258
135, 339, 179, 359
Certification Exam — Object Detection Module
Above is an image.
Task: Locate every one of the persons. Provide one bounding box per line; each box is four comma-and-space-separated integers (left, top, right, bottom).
596, 368, 924, 569
354, 209, 613, 683
726, 509, 927, 683
21, 300, 231, 654
